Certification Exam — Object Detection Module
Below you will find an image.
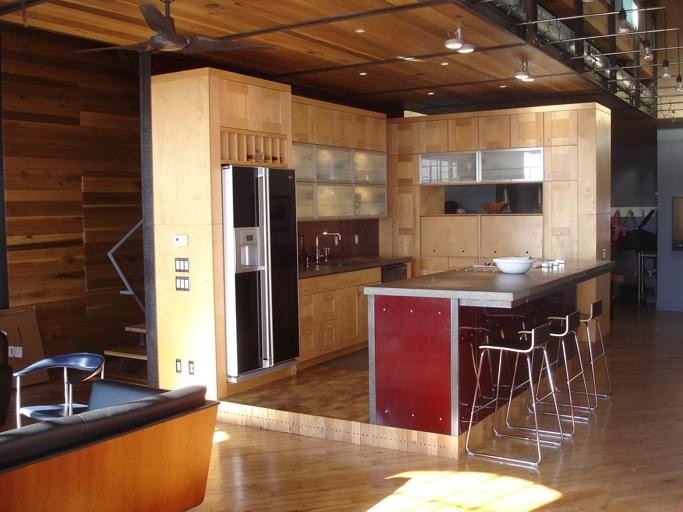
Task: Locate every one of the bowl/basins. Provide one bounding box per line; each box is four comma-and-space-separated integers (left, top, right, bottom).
492, 257, 533, 274
479, 202, 507, 214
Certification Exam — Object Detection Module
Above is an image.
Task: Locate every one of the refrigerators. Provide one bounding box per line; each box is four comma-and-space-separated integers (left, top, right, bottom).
220, 163, 298, 380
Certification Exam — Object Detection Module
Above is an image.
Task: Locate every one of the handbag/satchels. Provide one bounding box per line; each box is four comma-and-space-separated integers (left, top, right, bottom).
615, 225, 658, 251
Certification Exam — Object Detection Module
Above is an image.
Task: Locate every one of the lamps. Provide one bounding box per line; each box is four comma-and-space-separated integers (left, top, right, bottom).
612, 0, 683, 92
513, 55, 535, 82
443, 16, 475, 54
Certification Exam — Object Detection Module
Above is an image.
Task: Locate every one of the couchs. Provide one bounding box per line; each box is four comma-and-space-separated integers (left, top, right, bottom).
0, 377, 220, 512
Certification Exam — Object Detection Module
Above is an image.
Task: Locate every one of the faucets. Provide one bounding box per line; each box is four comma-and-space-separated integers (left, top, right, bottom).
315, 231, 341, 263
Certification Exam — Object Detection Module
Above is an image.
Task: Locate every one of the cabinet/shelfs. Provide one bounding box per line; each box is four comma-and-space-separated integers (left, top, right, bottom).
636, 248, 656, 308
298, 275, 339, 362
477, 146, 543, 184
388, 154, 419, 185
543, 148, 610, 182
542, 181, 610, 344
418, 152, 477, 185
390, 184, 445, 278
419, 216, 479, 257
510, 103, 611, 148
420, 256, 480, 280
480, 216, 541, 258
340, 268, 381, 357
292, 141, 386, 221
446, 115, 510, 150
290, 99, 341, 148
342, 110, 386, 154
150, 67, 288, 135
385, 120, 447, 153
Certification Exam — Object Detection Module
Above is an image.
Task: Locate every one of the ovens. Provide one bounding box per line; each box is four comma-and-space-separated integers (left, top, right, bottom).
380, 263, 408, 283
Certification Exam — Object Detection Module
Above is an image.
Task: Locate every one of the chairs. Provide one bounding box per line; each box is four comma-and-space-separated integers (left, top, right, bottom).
464, 323, 563, 467
535, 300, 612, 411
12, 353, 104, 430
505, 310, 591, 438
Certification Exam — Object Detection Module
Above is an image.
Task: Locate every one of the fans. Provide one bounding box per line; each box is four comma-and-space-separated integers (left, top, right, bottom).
69, 1, 275, 55
